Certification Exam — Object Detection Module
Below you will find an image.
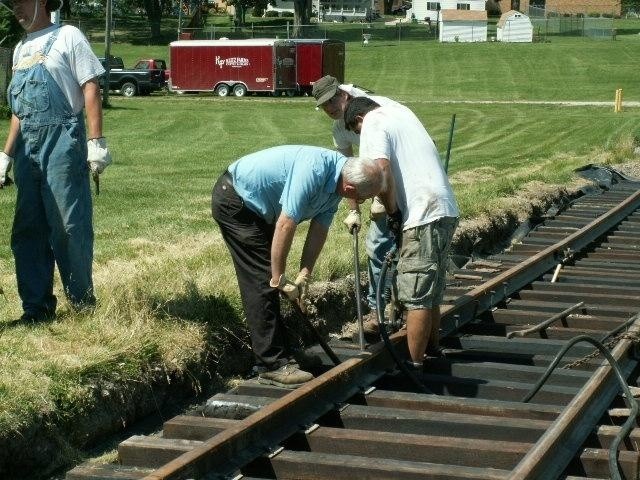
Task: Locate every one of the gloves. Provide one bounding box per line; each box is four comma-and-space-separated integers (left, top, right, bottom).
343, 209, 361, 235
270, 273, 311, 314
87, 136, 111, 174
0, 152, 15, 188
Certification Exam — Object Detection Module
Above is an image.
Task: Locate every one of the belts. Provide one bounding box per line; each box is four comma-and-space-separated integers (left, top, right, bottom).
223, 171, 233, 186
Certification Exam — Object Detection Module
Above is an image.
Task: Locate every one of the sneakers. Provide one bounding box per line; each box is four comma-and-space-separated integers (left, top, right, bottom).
10, 307, 57, 327
340, 314, 381, 344
258, 352, 322, 389
397, 354, 447, 371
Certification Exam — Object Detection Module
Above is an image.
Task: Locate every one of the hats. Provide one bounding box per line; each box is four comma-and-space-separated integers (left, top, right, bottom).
1, 0, 63, 11
312, 74, 339, 107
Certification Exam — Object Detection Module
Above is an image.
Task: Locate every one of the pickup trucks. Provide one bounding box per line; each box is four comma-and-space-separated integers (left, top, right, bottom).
97, 57, 165, 97
133, 59, 169, 81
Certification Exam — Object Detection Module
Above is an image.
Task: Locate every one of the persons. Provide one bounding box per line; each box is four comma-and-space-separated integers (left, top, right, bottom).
0, 0, 113, 322
212, 144, 383, 389
